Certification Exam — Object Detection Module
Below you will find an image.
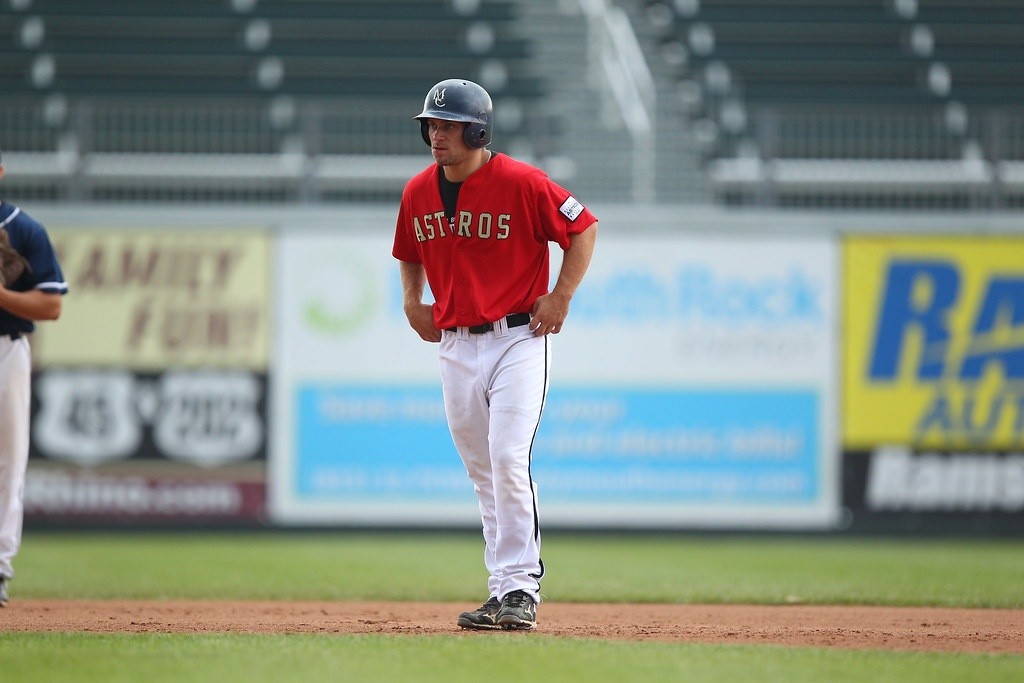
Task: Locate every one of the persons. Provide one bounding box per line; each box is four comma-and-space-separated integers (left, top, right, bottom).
393, 78, 599, 630
0, 164, 67, 606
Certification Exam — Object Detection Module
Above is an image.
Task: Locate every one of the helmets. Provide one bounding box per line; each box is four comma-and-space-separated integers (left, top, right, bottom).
413, 79, 492, 150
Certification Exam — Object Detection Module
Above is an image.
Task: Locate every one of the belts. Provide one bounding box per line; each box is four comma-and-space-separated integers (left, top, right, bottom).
446, 312, 530, 334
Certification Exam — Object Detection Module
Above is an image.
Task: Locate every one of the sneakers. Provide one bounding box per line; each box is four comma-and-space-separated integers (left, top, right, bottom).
497, 590, 536, 631
1, 576, 8, 608
457, 596, 514, 629
